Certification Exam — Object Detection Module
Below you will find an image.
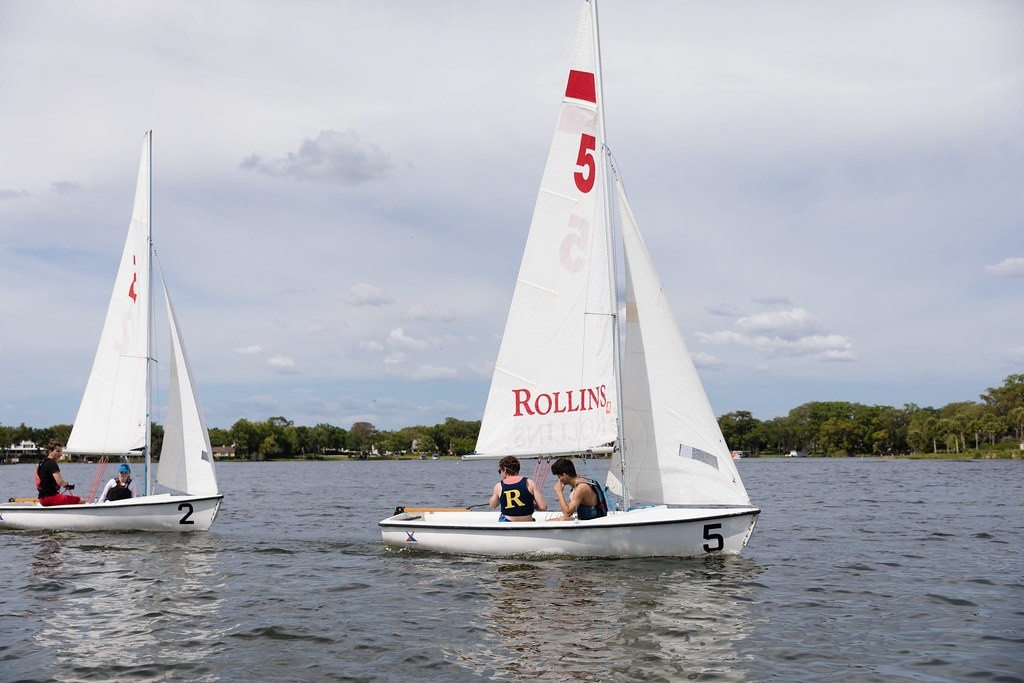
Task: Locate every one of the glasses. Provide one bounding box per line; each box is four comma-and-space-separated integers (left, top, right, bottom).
498, 468, 506, 474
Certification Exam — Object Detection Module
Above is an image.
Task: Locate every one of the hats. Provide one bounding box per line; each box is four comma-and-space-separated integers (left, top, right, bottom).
118, 464, 130, 473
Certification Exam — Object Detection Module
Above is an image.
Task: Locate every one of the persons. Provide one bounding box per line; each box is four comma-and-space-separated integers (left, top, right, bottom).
35, 441, 85, 506
98, 463, 137, 503
490, 456, 548, 522
545, 459, 609, 521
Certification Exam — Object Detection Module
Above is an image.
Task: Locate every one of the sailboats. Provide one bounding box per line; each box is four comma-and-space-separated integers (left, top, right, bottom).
0, 129, 225, 534
379, 0, 764, 558
